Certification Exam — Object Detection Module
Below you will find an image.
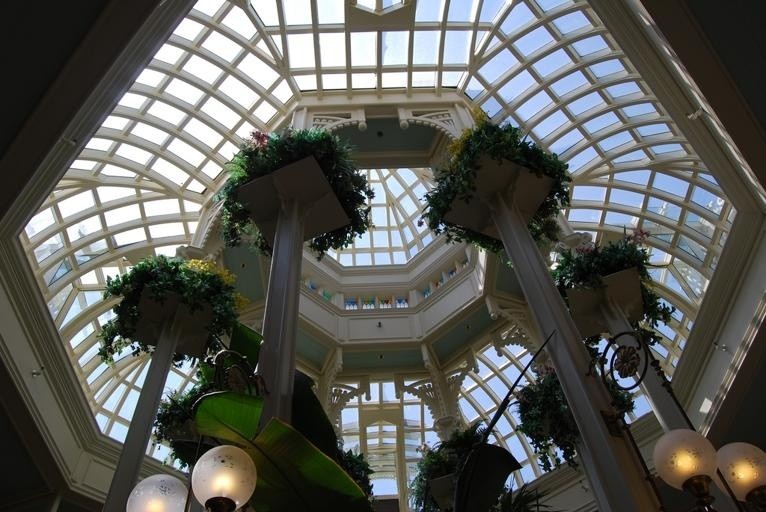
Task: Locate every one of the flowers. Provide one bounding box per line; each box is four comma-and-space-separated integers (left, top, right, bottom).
413, 103, 574, 249
92, 252, 252, 370
208, 114, 379, 263
547, 225, 678, 332
405, 415, 501, 511
509, 360, 637, 471
